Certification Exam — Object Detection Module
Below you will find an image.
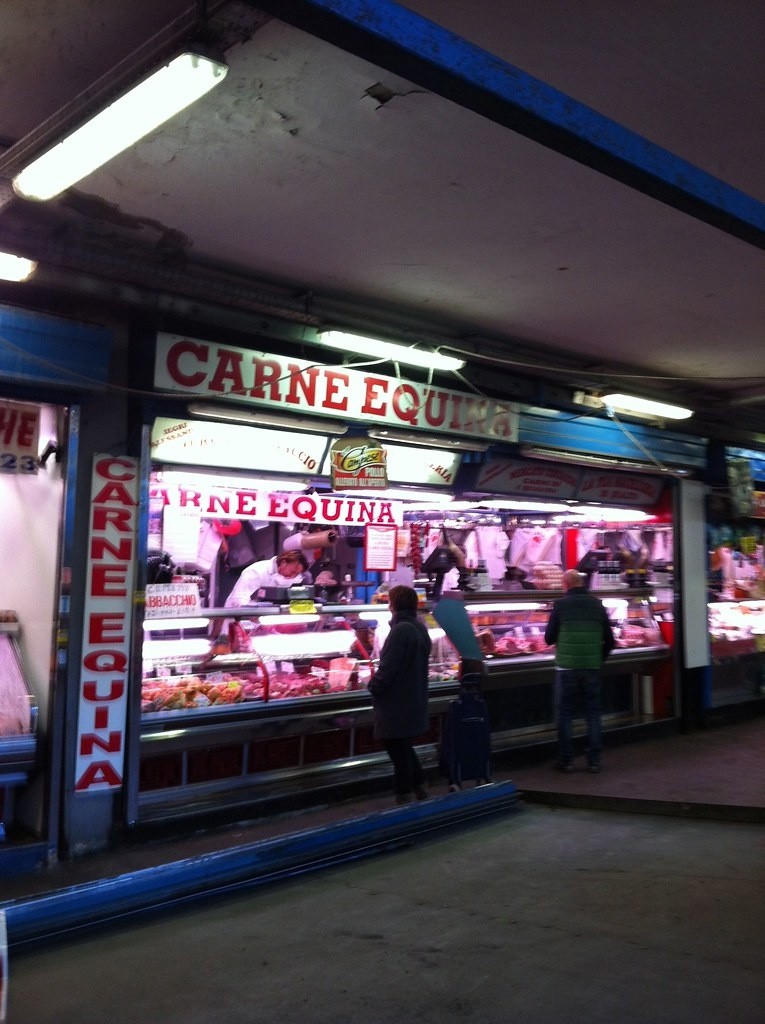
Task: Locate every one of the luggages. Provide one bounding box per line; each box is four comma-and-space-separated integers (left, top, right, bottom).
439, 669, 494, 791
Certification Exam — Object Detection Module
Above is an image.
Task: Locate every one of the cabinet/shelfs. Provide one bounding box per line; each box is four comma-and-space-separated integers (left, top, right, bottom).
124, 585, 676, 825
709, 588, 765, 697
0, 611, 39, 843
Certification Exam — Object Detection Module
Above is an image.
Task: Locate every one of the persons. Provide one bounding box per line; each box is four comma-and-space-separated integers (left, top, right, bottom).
218, 524, 339, 637
366, 585, 434, 804
543, 568, 617, 773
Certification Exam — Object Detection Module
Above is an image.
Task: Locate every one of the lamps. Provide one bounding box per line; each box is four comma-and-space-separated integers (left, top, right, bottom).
317, 325, 468, 371
598, 387, 697, 420
0, 247, 40, 283
12, 47, 230, 203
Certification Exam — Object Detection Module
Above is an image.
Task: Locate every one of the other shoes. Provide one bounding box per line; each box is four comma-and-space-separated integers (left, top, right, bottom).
564, 764, 576, 773
415, 785, 430, 800
395, 792, 411, 805
588, 765, 599, 773
